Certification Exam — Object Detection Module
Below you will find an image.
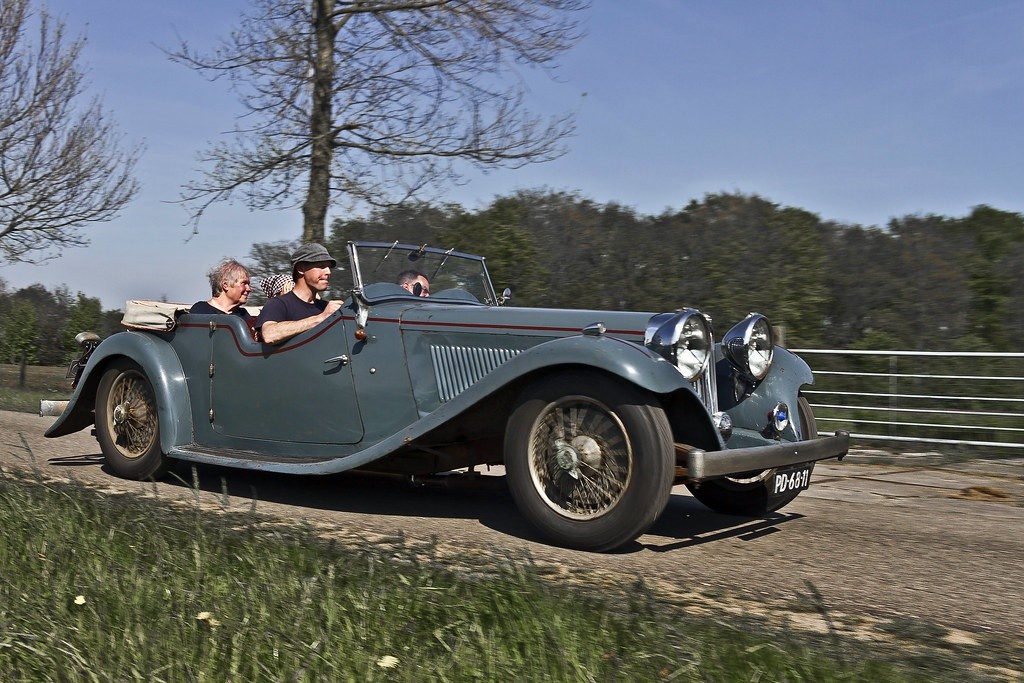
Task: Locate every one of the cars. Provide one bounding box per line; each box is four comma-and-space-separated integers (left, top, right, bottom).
45, 239, 850, 553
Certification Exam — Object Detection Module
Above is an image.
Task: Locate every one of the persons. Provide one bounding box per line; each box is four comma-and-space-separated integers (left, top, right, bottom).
397, 269, 430, 297
189, 260, 255, 330
255, 243, 344, 343
259, 274, 295, 298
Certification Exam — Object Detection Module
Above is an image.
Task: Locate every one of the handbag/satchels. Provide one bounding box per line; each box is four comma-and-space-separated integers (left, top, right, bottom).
121, 300, 192, 333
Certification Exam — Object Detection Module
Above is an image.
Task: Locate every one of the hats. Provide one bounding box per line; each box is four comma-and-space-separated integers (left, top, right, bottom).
290, 243, 336, 275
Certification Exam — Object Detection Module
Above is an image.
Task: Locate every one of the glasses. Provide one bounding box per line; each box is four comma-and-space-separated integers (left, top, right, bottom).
411, 284, 430, 294
